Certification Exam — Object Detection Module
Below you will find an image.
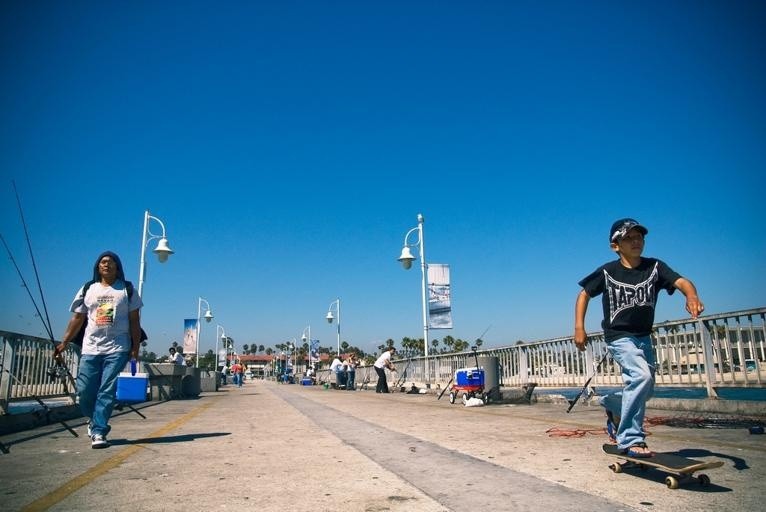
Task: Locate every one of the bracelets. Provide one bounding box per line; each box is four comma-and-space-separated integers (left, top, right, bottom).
62, 340, 72, 347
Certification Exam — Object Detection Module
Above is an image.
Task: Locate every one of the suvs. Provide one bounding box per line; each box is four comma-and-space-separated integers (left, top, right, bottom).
717, 361, 741, 373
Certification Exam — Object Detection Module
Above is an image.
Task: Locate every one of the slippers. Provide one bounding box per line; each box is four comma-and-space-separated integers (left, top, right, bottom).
604, 404, 619, 444
625, 442, 652, 457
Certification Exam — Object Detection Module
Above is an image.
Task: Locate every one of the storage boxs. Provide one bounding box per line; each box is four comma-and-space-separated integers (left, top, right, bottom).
454, 366, 484, 386
299, 376, 313, 386
330, 370, 347, 390
116, 358, 149, 403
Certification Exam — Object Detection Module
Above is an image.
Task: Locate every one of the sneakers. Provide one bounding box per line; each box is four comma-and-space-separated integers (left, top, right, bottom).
86, 418, 111, 449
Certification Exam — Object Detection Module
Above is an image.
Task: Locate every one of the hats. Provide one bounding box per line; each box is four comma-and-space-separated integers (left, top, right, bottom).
608, 217, 648, 247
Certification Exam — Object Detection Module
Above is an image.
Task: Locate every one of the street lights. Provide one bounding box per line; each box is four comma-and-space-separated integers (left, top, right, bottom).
136, 209, 173, 316
195, 294, 215, 367
326, 298, 341, 356
214, 323, 246, 376
398, 212, 433, 383
301, 325, 311, 367
262, 336, 297, 377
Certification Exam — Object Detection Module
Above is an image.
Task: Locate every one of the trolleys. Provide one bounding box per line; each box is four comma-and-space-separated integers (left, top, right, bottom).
446, 344, 490, 406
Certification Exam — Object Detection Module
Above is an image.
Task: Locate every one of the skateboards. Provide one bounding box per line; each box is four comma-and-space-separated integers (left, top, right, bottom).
602, 444, 673, 492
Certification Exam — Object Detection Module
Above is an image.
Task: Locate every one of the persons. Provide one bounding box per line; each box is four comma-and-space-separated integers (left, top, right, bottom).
164, 347, 187, 368
220, 359, 247, 388
330, 353, 359, 391
54, 251, 144, 448
374, 347, 397, 393
574, 218, 704, 458
306, 367, 316, 385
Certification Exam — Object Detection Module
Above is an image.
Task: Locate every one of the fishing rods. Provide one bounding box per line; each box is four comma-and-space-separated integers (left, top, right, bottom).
11, 179, 68, 397
438, 324, 489, 401
1, 364, 79, 438
0, 235, 78, 394
566, 350, 609, 413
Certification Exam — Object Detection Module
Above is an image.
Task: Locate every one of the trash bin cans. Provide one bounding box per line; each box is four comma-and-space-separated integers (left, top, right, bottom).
468, 357, 499, 401
180, 368, 200, 399
